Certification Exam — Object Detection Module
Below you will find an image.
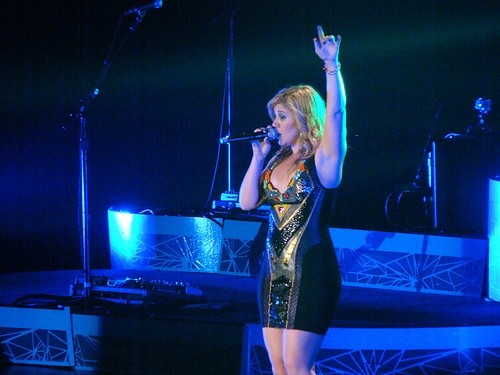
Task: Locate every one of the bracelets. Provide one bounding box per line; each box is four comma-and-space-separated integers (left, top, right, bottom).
322, 61, 341, 74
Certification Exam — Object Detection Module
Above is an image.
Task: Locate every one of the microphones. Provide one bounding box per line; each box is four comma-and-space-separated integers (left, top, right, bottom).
221, 128, 278, 143
128, 0, 163, 13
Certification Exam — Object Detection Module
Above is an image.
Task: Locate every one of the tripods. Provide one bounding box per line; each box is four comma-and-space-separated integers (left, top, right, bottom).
46, 13, 145, 310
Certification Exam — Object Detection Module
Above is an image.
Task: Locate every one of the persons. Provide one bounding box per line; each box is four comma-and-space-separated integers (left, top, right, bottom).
238, 26, 347, 375
